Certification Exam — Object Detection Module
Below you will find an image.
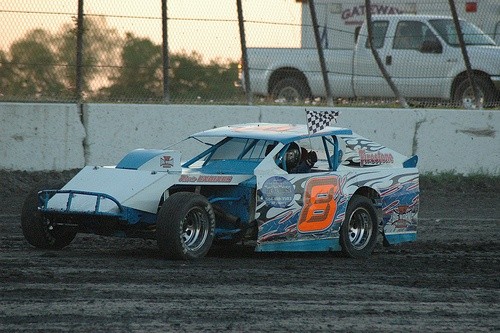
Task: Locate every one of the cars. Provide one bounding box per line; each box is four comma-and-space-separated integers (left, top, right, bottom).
20, 123, 419, 257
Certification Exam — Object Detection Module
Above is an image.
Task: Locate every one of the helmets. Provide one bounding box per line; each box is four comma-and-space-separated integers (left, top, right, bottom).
279, 141, 300, 167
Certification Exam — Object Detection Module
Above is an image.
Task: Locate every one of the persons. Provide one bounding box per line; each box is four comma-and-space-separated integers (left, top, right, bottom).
264, 139, 318, 176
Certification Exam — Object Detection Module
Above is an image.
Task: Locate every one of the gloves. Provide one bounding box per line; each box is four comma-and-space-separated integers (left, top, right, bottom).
306, 150, 317, 168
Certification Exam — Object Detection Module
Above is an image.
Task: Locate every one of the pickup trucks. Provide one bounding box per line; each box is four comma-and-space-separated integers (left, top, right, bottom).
240, 15, 500, 110
